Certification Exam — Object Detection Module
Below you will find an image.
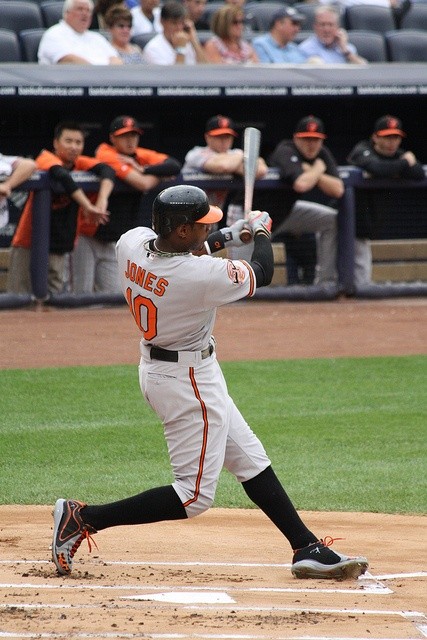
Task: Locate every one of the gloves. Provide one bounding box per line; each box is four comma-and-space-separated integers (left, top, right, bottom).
219, 219, 253, 248
247, 210, 273, 241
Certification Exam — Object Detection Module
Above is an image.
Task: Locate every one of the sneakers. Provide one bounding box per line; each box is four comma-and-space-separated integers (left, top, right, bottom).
51, 498, 98, 575
291, 536, 369, 581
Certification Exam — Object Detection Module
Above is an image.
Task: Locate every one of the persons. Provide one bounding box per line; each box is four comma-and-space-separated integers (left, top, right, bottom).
227, 115, 350, 284
10, 124, 116, 294
180, 114, 268, 219
62, 116, 181, 294
203, 7, 260, 66
37, 0, 121, 66
142, 0, 207, 66
104, 8, 142, 67
346, 117, 427, 286
128, 1, 166, 32
53, 184, 370, 579
183, 0, 212, 32
299, 7, 367, 66
255, 7, 307, 64
1, 151, 38, 291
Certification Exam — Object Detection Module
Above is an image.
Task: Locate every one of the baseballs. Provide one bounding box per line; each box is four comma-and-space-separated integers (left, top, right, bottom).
240, 126, 261, 242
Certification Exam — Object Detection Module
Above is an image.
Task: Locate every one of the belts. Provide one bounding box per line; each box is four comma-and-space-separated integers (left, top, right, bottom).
150, 343, 213, 362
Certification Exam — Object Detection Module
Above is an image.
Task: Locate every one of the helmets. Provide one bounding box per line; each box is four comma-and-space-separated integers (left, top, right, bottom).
152, 184, 224, 233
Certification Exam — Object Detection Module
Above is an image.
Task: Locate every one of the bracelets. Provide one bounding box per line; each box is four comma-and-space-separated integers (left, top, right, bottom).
175, 47, 187, 55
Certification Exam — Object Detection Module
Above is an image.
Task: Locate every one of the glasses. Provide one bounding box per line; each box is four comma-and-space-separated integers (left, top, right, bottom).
232, 19, 246, 23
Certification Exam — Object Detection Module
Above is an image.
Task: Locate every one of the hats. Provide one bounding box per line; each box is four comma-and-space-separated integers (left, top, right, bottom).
295, 114, 327, 140
374, 115, 407, 138
269, 6, 307, 24
205, 114, 240, 137
109, 115, 144, 137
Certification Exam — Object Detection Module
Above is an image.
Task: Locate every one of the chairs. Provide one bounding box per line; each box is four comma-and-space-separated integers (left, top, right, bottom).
1, 29, 20, 61
21, 28, 46, 62
347, 30, 385, 64
41, 2, 63, 26
346, 6, 395, 32
386, 30, 427, 61
1, 2, 43, 30
91, 1, 343, 50
398, 1, 427, 28
